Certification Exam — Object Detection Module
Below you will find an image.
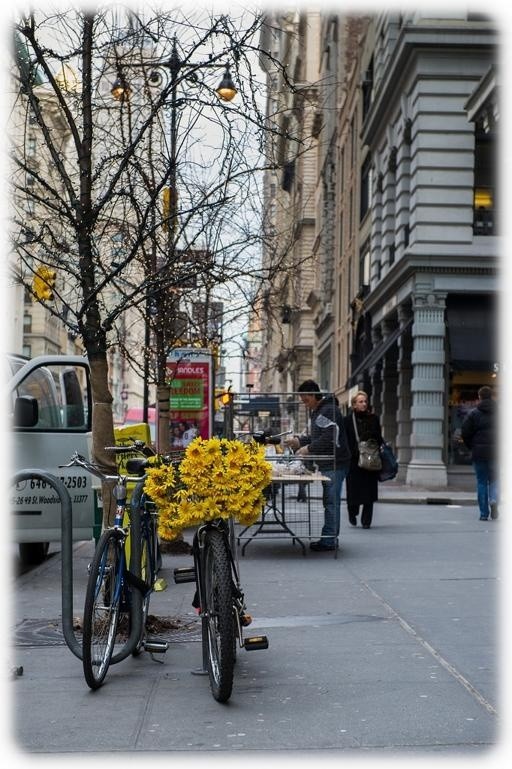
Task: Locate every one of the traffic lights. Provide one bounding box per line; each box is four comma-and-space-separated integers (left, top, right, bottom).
31, 265, 57, 302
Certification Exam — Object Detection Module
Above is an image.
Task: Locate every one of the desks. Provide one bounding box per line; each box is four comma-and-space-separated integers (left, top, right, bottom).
238, 472, 330, 557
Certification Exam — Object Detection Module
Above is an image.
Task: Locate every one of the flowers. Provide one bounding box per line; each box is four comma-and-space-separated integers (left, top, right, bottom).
144, 436, 273, 541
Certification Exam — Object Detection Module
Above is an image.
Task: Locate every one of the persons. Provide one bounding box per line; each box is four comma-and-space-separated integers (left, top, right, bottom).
238, 423, 252, 444
254, 426, 310, 507
170, 426, 182, 447
212, 428, 235, 442
281, 378, 352, 554
342, 390, 389, 531
178, 421, 201, 448
456, 386, 499, 522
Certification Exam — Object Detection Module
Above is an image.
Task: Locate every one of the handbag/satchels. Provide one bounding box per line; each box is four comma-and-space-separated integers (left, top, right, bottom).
381, 447, 399, 481
359, 442, 382, 470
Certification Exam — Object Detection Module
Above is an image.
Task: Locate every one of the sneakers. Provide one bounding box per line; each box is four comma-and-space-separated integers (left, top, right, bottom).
310, 541, 339, 550
490, 500, 498, 520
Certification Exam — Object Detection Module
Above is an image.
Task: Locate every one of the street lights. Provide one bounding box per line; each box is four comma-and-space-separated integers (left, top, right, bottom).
110, 32, 238, 459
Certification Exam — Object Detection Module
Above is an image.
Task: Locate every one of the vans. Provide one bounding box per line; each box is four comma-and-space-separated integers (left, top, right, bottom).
7, 347, 98, 567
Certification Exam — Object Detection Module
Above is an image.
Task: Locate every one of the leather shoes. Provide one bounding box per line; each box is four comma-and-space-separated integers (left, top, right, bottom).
349, 511, 357, 526
361, 519, 370, 528
